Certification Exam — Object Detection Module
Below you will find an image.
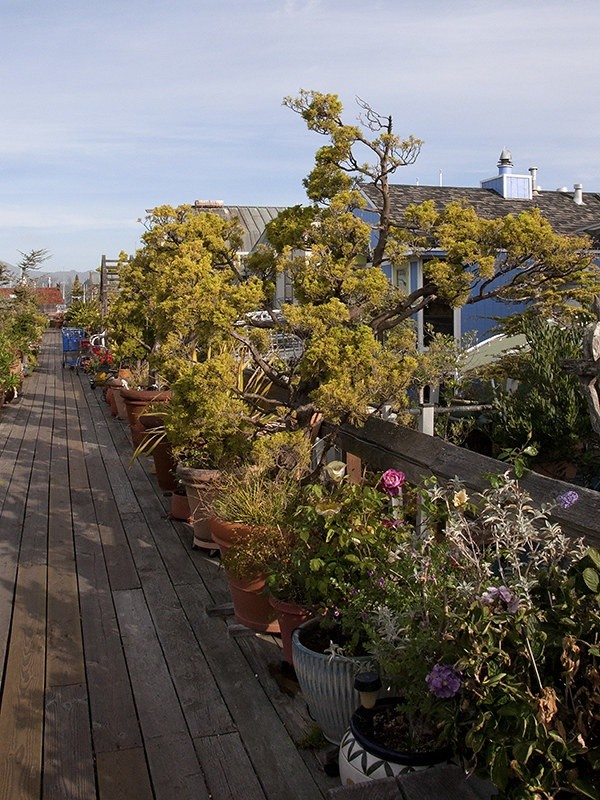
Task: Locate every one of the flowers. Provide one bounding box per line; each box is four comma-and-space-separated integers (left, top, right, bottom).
265, 431, 600, 800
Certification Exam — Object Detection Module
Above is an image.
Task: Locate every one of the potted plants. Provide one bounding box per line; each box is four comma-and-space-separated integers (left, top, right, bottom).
108, 335, 313, 632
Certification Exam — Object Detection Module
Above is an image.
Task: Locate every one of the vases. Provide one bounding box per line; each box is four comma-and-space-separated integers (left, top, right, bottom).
339, 697, 458, 785
291, 618, 387, 746
269, 593, 319, 664
